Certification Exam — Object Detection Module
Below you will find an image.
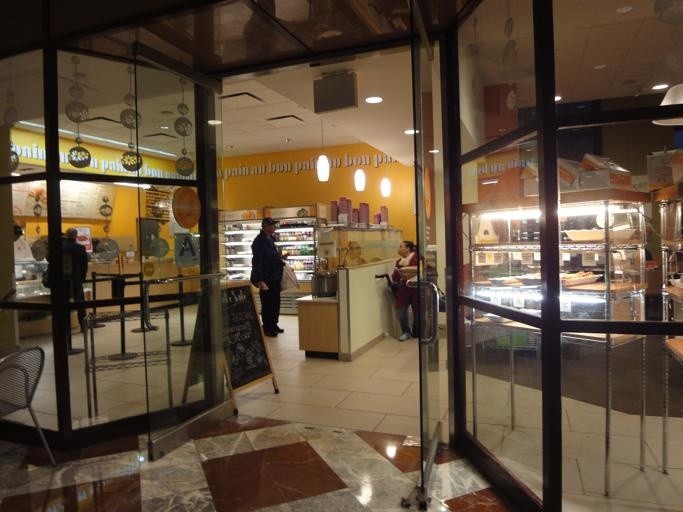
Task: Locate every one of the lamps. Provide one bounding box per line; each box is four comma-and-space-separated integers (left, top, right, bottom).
315, 118, 393, 198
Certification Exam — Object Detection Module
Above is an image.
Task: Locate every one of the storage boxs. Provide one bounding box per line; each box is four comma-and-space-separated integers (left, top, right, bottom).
521, 148, 683, 196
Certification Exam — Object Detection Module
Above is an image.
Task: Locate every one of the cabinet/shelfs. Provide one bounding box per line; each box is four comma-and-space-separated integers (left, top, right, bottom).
15, 186, 683, 496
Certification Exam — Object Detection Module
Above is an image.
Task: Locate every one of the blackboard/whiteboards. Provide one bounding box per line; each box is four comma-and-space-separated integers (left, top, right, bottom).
216, 279, 274, 395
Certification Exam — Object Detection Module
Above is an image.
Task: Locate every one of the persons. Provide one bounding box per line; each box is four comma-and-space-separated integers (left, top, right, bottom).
42, 227, 91, 332
13, 220, 35, 281
390, 240, 420, 341
250, 216, 290, 337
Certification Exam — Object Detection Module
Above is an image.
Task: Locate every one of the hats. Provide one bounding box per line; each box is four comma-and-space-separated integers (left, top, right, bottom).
260, 217, 280, 229
13, 224, 24, 236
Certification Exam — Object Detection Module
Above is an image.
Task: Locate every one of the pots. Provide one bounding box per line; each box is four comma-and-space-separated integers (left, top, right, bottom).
310, 272, 336, 296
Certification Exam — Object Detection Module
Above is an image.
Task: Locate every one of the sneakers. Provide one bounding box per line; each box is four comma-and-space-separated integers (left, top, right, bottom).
263, 324, 284, 338
398, 332, 410, 342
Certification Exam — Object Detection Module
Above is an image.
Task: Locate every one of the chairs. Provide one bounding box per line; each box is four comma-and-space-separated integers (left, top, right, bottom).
0, 344, 58, 467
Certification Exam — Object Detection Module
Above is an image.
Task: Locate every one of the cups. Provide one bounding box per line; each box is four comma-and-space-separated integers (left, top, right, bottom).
331, 197, 389, 224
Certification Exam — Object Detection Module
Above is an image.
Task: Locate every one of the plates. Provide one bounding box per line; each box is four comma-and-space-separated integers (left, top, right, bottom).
484, 229, 637, 323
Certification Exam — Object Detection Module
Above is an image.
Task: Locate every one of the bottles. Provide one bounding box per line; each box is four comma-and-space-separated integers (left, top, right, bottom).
278, 229, 314, 271
